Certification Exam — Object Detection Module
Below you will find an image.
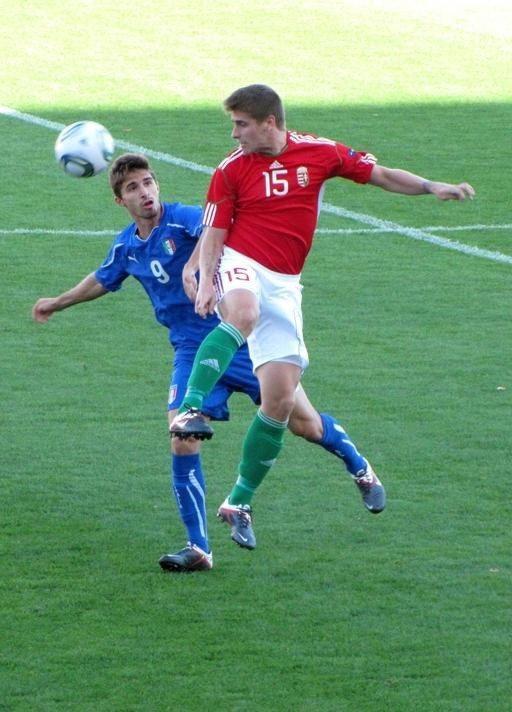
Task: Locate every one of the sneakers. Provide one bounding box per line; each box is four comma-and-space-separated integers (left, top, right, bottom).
168, 408, 214, 442
216, 495, 257, 551
158, 541, 213, 571
350, 455, 385, 515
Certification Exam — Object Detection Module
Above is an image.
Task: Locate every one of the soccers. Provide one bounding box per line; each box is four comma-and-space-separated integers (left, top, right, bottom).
55, 120, 114, 177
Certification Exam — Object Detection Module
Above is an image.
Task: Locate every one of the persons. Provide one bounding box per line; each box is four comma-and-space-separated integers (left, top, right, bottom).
32, 152, 388, 573
165, 83, 479, 550
